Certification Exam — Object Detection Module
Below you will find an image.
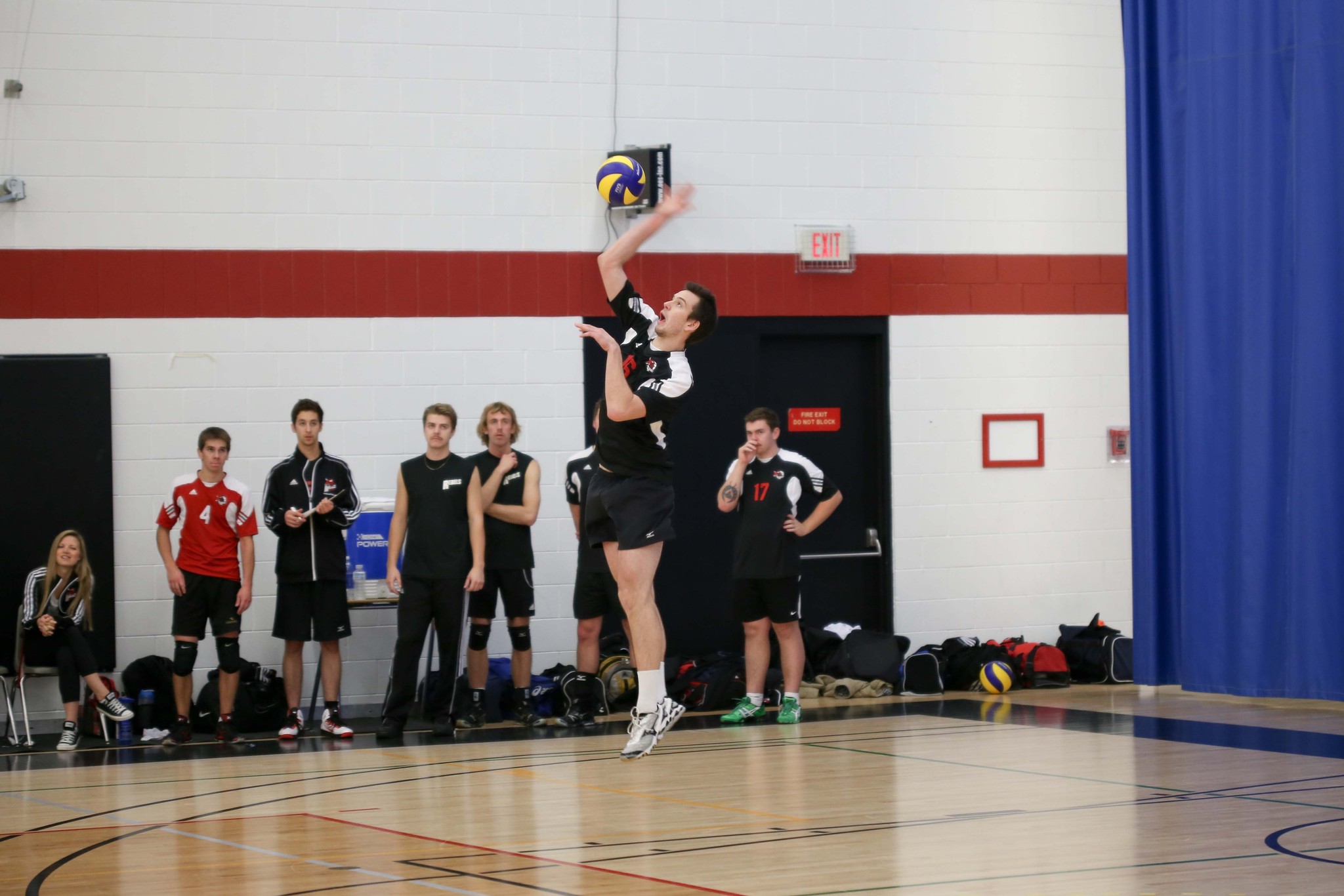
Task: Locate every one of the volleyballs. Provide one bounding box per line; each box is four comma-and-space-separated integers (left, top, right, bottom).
980, 661, 1014, 693
598, 154, 644, 206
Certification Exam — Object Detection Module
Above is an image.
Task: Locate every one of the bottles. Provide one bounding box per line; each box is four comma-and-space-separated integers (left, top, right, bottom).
138, 689, 155, 734
118, 697, 134, 745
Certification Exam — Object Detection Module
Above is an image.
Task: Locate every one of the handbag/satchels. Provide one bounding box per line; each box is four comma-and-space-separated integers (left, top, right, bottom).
798, 622, 1071, 695
673, 650, 782, 711
419, 657, 639, 717
1055, 613, 1134, 685
195, 658, 286, 730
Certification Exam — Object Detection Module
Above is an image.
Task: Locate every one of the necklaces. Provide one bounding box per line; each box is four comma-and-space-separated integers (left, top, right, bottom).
424, 452, 451, 470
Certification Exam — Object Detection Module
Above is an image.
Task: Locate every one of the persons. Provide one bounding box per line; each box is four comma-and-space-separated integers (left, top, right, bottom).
717, 408, 843, 724
545, 397, 639, 728
577, 183, 718, 760
21, 529, 135, 750
457, 403, 550, 728
376, 404, 487, 739
156, 426, 260, 746
260, 399, 362, 741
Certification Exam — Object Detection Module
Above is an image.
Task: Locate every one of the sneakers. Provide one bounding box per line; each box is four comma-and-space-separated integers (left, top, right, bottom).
216, 714, 243, 744
554, 698, 596, 727
513, 700, 546, 727
278, 707, 304, 740
171, 715, 192, 743
320, 707, 354, 737
56, 721, 80, 750
96, 691, 134, 721
620, 697, 685, 761
777, 696, 802, 724
456, 703, 486, 729
720, 696, 767, 723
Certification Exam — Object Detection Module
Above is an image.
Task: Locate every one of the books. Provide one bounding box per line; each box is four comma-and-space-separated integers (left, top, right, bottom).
296, 488, 344, 521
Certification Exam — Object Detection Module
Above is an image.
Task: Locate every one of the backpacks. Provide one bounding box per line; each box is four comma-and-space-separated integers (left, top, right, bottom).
120, 654, 192, 737
79, 676, 121, 737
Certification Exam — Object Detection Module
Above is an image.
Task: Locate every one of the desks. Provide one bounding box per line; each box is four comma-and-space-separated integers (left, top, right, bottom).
306, 598, 436, 729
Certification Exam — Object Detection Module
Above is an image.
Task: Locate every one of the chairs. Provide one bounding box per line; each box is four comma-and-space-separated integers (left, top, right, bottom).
0, 658, 22, 749
2, 605, 112, 748
